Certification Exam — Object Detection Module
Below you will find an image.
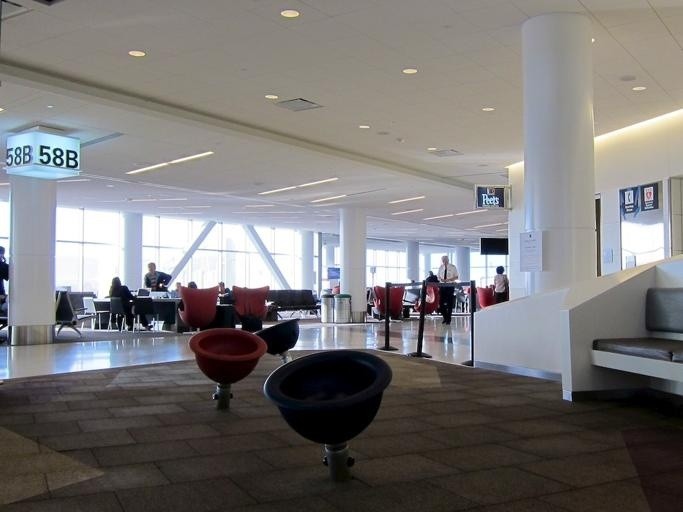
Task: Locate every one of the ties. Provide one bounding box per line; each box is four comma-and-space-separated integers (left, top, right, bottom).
443, 266, 448, 281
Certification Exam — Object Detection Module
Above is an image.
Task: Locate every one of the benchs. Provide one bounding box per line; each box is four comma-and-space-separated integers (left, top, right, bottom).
268, 290, 321, 319
592, 288, 683, 384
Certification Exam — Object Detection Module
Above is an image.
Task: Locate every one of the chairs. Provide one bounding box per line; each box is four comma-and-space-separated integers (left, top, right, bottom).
56, 286, 270, 335
371, 284, 498, 324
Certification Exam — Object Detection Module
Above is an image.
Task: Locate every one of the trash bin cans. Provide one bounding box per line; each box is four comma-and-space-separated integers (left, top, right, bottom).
320, 294, 351, 323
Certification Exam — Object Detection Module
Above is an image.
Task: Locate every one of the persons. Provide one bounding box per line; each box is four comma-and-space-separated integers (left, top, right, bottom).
426, 271, 440, 283
109, 276, 153, 331
438, 256, 459, 326
0, 246, 9, 295
493, 266, 509, 303
143, 263, 173, 292
170, 282, 231, 311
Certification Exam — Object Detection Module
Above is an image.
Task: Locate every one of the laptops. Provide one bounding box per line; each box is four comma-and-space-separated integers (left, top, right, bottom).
137, 288, 151, 298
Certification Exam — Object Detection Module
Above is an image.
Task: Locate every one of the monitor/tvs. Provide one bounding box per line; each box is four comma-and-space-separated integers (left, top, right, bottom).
481, 237, 508, 256
